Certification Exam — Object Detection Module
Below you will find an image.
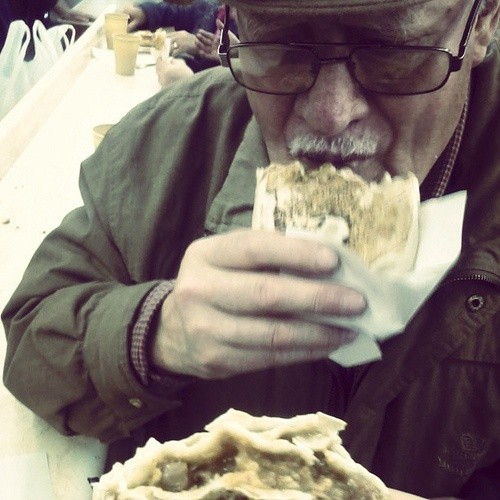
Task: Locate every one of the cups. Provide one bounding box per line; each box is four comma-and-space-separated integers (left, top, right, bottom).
94, 124, 113, 152
106, 13, 130, 49
114, 35, 142, 76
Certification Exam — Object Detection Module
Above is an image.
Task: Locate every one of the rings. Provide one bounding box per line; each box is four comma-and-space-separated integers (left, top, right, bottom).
173, 41, 178, 50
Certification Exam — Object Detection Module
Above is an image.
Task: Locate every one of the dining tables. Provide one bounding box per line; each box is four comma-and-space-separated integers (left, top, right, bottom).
0, 0, 175, 500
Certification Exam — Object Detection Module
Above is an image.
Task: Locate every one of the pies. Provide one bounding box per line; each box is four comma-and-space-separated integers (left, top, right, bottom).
263, 161, 422, 285
89, 408, 389, 500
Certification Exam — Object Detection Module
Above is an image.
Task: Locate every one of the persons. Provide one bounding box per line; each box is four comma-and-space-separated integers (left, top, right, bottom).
110, 0, 239, 66
0, 1, 500, 500
192, 17, 244, 67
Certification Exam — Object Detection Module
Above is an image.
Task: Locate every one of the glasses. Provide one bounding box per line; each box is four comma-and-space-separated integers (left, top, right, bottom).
217, 0, 482, 96
